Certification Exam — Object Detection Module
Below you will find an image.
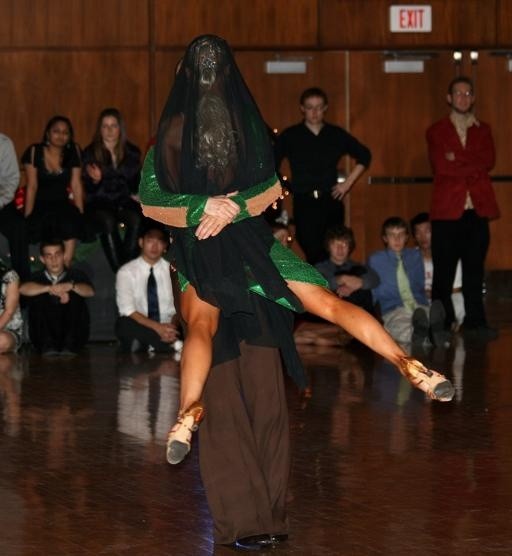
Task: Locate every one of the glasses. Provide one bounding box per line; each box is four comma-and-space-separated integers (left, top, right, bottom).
452, 90, 471, 97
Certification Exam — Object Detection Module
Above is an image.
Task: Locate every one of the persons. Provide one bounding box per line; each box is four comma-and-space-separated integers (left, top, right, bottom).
427, 78, 502, 343
138, 55, 310, 552
155, 35, 455, 466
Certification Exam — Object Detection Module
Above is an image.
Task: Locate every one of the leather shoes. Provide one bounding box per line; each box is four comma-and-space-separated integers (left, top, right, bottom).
222, 534, 289, 552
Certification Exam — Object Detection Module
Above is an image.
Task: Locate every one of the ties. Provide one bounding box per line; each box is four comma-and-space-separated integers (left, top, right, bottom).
147, 267, 160, 322
395, 254, 418, 312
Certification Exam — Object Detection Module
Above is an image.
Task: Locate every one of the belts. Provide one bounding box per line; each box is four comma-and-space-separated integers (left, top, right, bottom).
306, 188, 327, 200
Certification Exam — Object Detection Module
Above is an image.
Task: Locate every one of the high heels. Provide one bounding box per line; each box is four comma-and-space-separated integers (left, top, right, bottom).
399, 355, 456, 403
163, 402, 207, 466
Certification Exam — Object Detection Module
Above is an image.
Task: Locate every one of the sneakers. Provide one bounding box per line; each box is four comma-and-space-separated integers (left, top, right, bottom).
462, 326, 499, 341
41, 336, 82, 357
410, 307, 429, 347
429, 299, 447, 351
